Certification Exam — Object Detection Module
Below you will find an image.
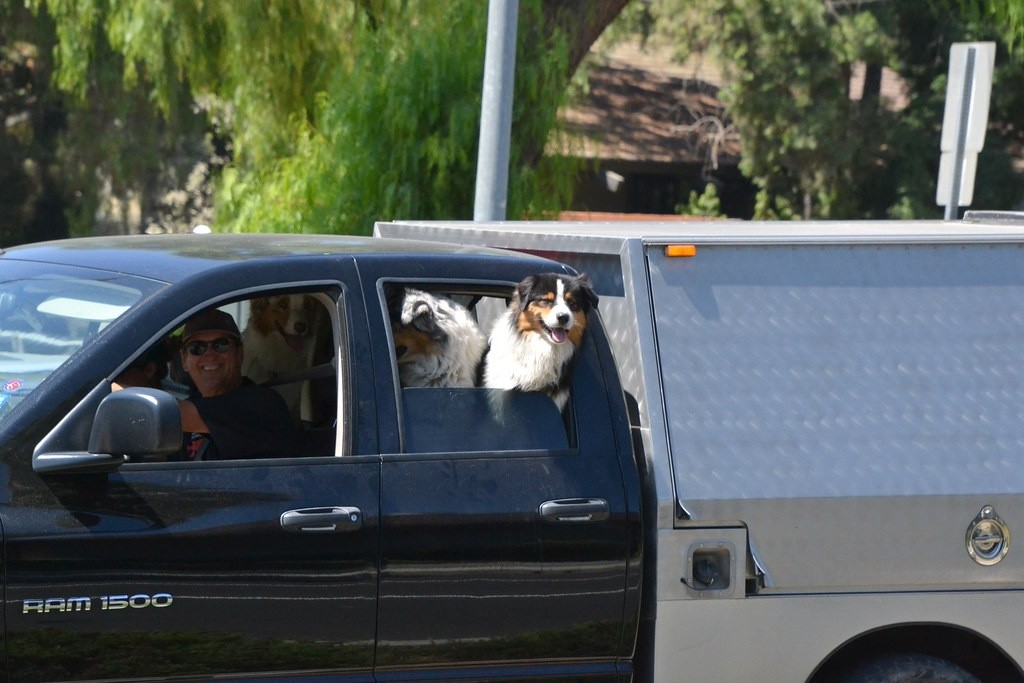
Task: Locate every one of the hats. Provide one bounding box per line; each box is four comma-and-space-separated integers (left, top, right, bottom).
182, 309, 241, 342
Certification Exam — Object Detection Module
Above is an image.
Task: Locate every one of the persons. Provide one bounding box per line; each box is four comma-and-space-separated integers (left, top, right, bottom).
111, 308, 301, 461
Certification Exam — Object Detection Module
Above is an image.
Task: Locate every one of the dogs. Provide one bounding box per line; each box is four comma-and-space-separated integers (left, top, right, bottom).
240, 292, 319, 427
482, 272, 599, 429
381, 287, 489, 437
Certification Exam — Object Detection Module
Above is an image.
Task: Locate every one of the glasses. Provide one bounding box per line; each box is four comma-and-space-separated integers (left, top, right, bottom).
184, 336, 239, 356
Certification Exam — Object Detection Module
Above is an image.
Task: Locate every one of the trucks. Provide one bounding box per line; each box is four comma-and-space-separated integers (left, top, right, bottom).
0, 220, 1024, 683
